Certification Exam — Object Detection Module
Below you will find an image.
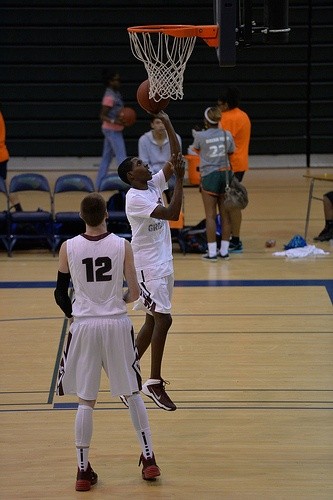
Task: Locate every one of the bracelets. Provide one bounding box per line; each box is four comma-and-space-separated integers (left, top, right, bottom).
111, 119, 115, 124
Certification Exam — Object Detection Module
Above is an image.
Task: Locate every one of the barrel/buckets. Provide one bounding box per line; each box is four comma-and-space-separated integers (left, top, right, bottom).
182, 155, 200, 184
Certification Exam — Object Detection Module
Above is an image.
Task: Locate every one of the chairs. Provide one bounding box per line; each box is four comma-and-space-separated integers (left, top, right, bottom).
0, 174, 135, 257
158, 174, 186, 254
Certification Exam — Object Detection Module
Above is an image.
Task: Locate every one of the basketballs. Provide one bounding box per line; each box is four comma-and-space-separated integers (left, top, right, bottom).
117, 108, 136, 126
137, 80, 170, 112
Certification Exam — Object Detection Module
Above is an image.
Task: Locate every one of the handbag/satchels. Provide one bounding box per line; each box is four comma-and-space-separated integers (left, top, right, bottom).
224, 177, 248, 209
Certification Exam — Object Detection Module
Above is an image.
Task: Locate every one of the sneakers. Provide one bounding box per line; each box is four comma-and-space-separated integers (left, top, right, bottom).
120, 395, 129, 408
141, 377, 177, 411
75, 461, 98, 491
138, 451, 160, 479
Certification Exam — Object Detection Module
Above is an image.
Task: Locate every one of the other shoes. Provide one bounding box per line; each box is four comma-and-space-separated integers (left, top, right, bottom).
314, 231, 333, 242
228, 239, 243, 253
216, 252, 229, 259
201, 253, 217, 261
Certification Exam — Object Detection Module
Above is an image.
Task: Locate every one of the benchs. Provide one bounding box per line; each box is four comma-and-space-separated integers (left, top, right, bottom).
0, 0, 333, 158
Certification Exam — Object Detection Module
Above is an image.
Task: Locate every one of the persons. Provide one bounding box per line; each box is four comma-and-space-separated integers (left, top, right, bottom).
0, 110, 19, 212
313, 191, 333, 242
191, 105, 235, 262
117, 109, 186, 411
53, 193, 160, 492
217, 86, 251, 253
94, 72, 128, 193
